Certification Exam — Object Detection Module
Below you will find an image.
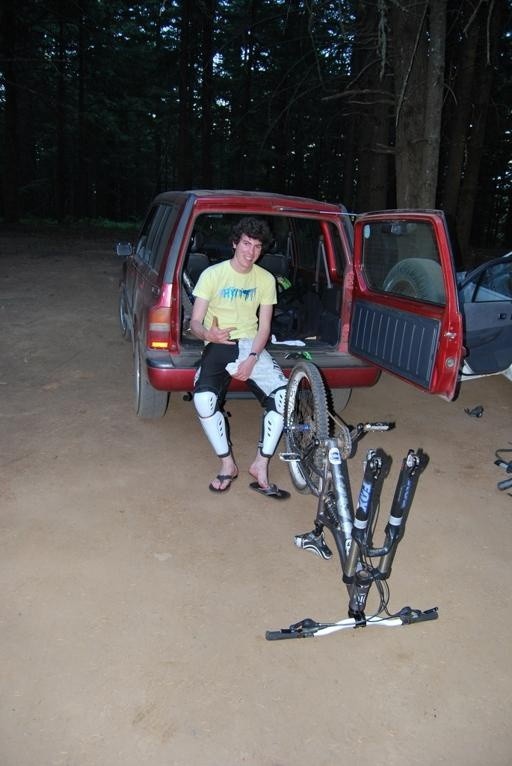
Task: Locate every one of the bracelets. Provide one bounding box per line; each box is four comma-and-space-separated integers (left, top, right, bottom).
248, 351, 260, 362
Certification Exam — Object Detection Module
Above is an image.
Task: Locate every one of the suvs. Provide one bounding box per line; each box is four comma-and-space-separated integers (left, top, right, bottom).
114, 187, 462, 418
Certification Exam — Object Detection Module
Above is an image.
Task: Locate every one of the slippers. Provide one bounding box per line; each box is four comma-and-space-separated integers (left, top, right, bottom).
209, 475, 237, 492
249, 482, 291, 499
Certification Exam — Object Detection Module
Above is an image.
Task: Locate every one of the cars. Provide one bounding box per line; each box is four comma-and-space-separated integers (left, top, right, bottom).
458, 252, 512, 386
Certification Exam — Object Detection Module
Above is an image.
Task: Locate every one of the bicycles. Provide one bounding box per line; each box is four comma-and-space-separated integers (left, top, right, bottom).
260, 362, 444, 640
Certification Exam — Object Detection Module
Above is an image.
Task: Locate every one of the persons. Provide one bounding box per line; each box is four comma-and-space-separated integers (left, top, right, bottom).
189, 219, 294, 500
